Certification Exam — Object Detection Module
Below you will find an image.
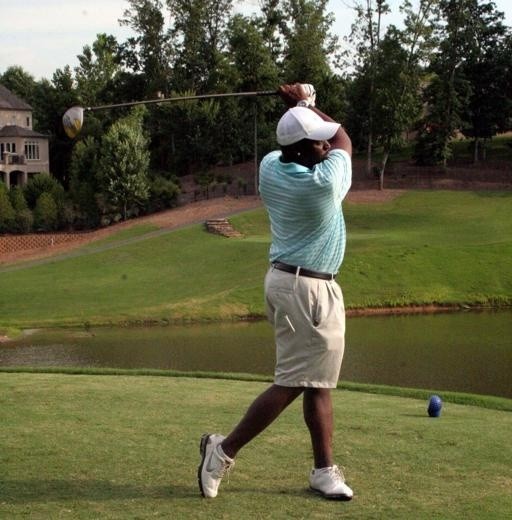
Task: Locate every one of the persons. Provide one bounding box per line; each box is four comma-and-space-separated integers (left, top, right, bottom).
198, 82, 354, 500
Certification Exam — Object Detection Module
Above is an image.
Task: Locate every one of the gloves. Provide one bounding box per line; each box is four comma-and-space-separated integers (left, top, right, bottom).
278, 82, 316, 109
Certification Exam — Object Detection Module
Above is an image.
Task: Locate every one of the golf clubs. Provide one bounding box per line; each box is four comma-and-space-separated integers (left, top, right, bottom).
63, 88, 279, 138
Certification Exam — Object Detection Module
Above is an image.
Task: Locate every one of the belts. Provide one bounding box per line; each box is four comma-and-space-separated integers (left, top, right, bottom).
270, 260, 338, 280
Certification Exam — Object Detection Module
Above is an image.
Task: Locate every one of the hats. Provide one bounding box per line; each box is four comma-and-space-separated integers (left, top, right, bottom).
276, 106, 342, 147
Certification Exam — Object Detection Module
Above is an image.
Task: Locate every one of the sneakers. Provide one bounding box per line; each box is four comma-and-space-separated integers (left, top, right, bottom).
309, 464, 354, 501
198, 433, 235, 498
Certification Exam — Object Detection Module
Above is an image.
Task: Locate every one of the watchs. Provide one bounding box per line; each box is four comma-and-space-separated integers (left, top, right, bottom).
296, 99, 314, 108
